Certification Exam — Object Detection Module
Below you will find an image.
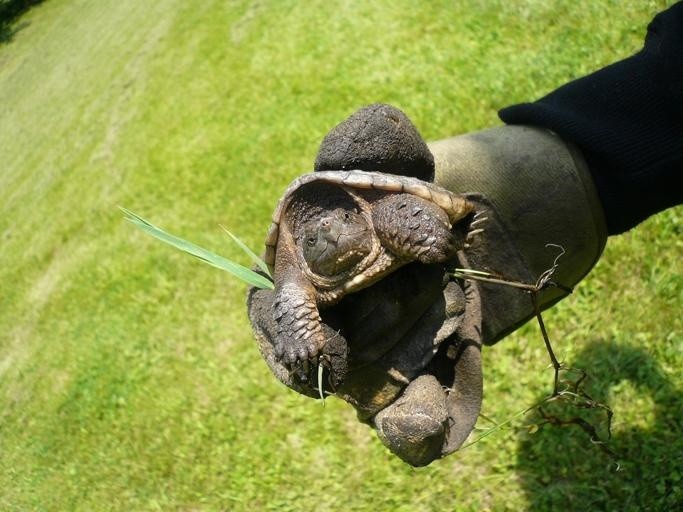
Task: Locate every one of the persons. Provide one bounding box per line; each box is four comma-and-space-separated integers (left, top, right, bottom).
248, 0, 682, 467
